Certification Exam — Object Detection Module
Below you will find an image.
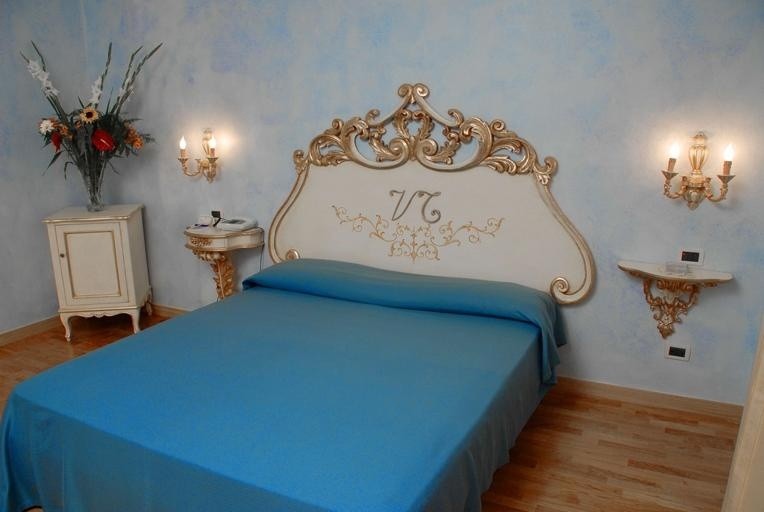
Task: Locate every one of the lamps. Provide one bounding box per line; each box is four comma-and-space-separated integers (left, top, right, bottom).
661, 129, 735, 211
177, 128, 219, 185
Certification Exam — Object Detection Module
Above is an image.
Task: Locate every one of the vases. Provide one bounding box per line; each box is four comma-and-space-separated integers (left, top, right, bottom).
78, 167, 105, 213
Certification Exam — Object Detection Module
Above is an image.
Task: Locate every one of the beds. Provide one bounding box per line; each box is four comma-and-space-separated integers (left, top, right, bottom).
0, 80, 596, 512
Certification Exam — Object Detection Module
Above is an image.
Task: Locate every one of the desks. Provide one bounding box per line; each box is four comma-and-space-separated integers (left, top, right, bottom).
182, 224, 264, 302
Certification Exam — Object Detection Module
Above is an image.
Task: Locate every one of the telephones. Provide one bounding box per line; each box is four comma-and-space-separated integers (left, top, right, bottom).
217, 216, 259, 232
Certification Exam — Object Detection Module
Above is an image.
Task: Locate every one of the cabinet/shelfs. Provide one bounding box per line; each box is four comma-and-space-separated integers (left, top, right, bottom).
39, 205, 153, 342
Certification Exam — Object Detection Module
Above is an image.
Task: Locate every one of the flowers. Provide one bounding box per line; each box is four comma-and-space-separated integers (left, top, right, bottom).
18, 40, 162, 204
